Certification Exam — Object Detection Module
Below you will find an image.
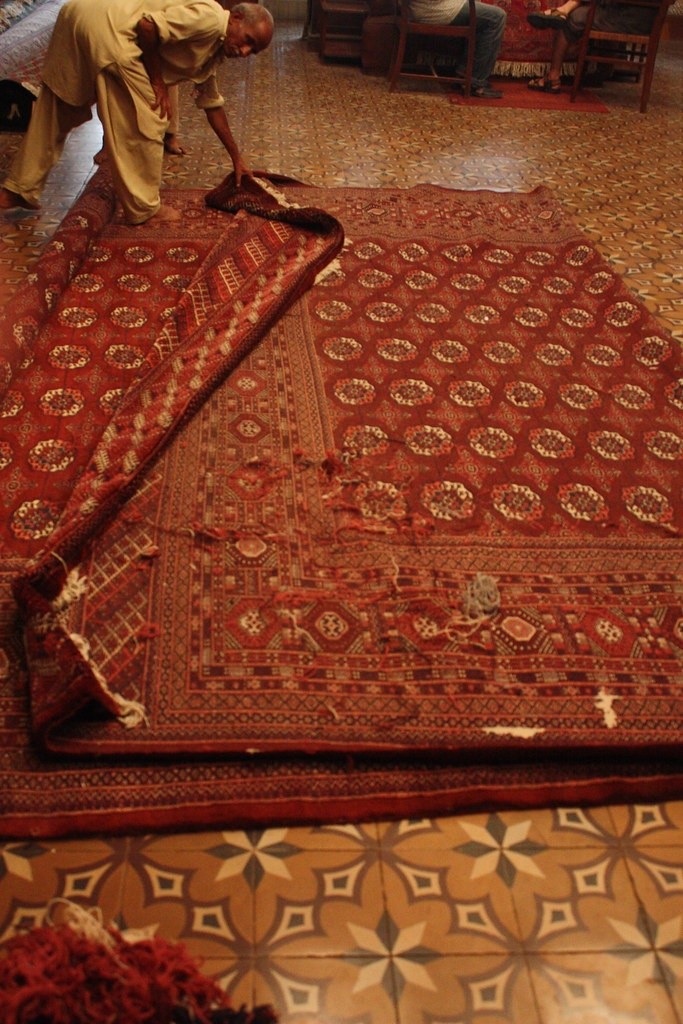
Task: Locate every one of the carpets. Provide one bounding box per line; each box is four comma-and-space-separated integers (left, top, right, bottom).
0, 146, 683, 841
437, 73, 609, 113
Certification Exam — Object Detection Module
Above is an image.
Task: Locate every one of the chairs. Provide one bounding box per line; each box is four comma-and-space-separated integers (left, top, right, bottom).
389, 0, 476, 95
571, 0, 675, 112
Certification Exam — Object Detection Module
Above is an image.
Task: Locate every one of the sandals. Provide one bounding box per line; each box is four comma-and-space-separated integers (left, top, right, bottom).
528, 73, 561, 94
526, 7, 568, 30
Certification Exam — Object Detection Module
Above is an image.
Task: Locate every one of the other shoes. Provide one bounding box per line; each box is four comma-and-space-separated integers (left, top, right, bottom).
473, 86, 504, 99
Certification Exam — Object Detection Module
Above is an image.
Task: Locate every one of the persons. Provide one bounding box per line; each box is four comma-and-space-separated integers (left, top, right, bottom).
402, 0, 507, 98
527, 0, 672, 95
1, 1, 276, 223
